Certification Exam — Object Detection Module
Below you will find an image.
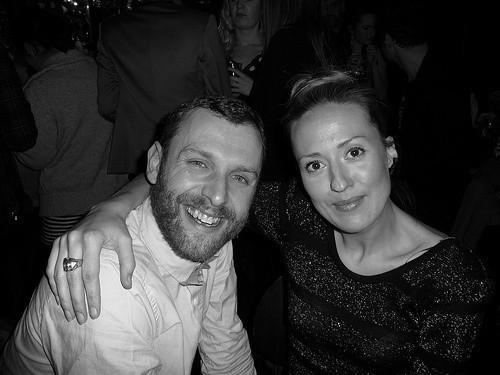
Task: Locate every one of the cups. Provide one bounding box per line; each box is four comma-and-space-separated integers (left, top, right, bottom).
228, 63, 242, 97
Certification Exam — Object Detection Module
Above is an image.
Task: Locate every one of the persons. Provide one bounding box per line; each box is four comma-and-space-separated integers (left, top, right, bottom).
127, 66, 500, 375
0, 0, 498, 246
0, 92, 268, 375
